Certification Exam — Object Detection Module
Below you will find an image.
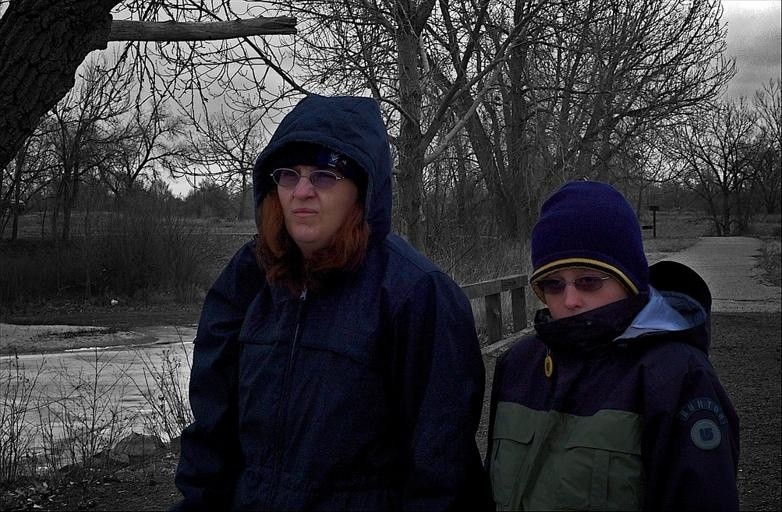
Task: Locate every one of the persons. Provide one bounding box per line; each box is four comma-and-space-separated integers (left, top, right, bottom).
486, 180, 741, 512
168, 94, 495, 511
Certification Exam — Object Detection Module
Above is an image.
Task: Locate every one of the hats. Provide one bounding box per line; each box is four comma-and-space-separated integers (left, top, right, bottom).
530, 181, 650, 304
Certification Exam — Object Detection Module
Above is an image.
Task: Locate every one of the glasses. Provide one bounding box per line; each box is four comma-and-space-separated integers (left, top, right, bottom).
534, 275, 613, 295
269, 167, 347, 190
